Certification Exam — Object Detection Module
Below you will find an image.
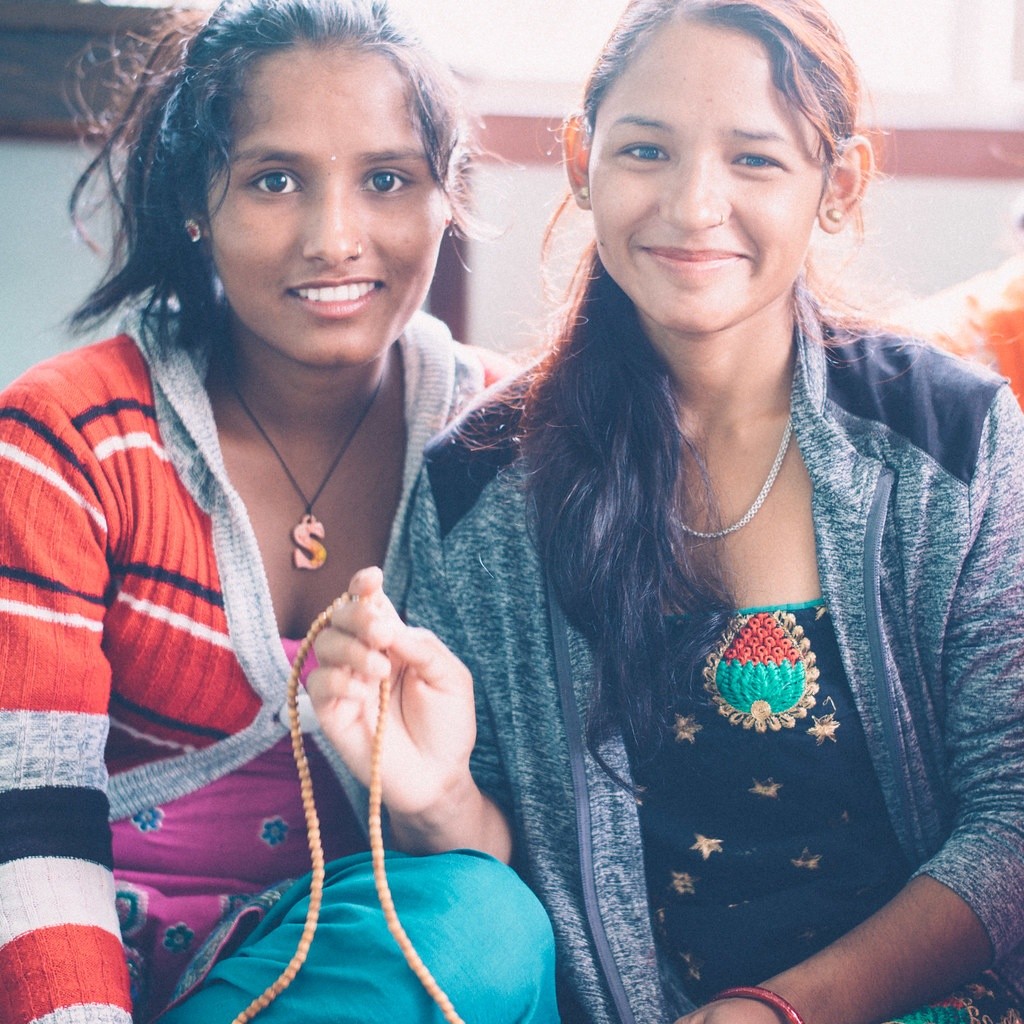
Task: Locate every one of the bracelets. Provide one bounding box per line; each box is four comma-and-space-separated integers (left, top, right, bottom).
707, 986, 805, 1024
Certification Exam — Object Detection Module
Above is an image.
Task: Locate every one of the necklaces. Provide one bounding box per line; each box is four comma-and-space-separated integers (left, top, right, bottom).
665, 407, 795, 540
218, 347, 390, 571
226, 592, 469, 1024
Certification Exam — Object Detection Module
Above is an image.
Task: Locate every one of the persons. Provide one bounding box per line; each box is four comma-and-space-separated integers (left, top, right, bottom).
286, 4, 1023, 1024
0, 2, 560, 1024
